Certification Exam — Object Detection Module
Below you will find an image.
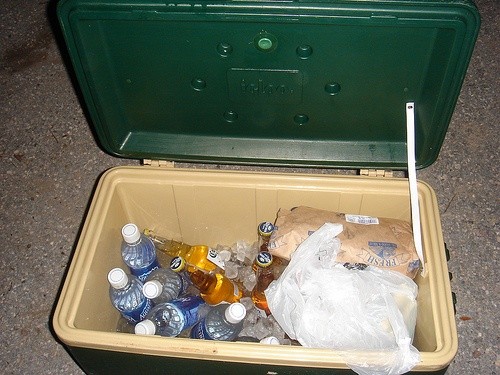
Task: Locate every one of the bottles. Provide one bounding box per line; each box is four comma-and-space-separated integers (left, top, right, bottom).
107, 267, 154, 326
233, 336, 280, 345
142, 229, 246, 287
142, 268, 190, 305
134, 296, 211, 337
184, 303, 246, 341
121, 223, 161, 282
251, 252, 277, 317
252, 221, 281, 275
170, 256, 247, 305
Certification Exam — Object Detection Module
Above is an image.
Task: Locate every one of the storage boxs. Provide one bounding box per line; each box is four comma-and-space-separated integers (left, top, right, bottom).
45, 1, 482, 375
